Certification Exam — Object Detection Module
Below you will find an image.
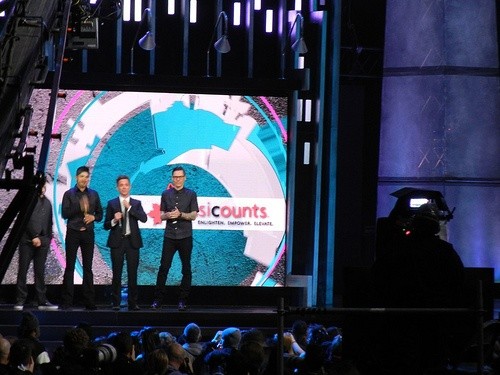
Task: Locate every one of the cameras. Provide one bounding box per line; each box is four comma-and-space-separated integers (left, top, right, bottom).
83, 343, 117, 364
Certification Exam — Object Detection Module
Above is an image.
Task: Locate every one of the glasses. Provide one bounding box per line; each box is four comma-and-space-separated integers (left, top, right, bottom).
172, 174, 185, 180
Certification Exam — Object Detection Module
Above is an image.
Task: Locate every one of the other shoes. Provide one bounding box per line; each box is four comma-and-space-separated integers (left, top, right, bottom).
151, 301, 160, 311
38, 300, 59, 310
79, 304, 97, 310
179, 302, 187, 311
112, 304, 120, 310
128, 304, 141, 310
14, 303, 23, 310
61, 303, 71, 309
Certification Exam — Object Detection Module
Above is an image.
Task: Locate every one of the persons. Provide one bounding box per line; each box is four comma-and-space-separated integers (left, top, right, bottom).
59, 166, 104, 310
0, 309, 357, 375
104, 176, 147, 310
152, 167, 199, 310
15, 184, 54, 306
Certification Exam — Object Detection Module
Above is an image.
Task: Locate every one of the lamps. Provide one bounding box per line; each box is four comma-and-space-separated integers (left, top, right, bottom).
280, 13, 308, 79
130, 7, 156, 73
207, 11, 230, 77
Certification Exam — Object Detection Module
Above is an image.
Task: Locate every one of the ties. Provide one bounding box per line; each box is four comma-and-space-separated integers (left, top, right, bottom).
122, 207, 128, 236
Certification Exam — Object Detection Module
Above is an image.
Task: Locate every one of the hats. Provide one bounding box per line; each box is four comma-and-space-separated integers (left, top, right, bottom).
21, 311, 40, 328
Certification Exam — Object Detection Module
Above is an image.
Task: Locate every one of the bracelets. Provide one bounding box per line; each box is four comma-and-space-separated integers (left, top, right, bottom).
178, 212, 182, 218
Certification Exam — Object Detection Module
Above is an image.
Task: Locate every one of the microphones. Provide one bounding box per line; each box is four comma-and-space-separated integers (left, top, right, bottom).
119, 219, 122, 227
